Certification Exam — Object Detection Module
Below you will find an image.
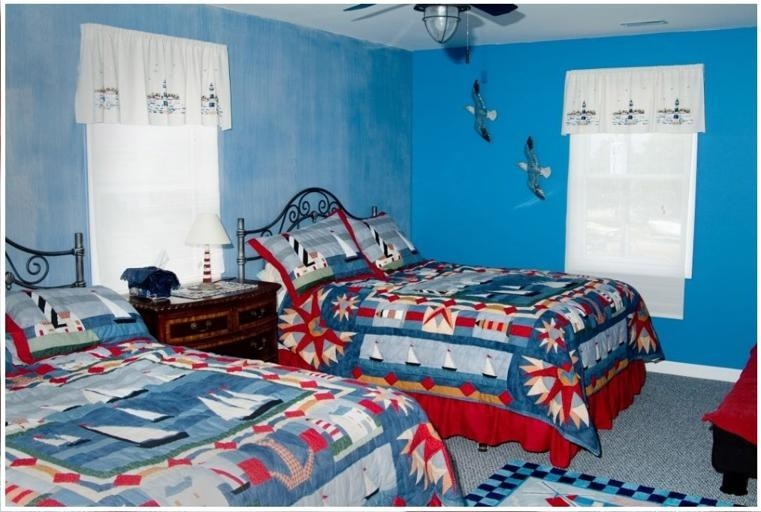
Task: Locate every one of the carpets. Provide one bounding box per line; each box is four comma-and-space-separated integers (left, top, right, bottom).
463, 459, 750, 507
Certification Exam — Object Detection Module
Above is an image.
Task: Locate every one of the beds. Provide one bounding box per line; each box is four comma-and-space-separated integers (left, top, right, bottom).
237, 188, 638, 469
5, 232, 417, 506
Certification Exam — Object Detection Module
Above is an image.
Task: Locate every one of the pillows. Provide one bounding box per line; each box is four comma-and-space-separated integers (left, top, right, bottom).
343, 212, 424, 278
5, 286, 157, 367
248, 209, 390, 306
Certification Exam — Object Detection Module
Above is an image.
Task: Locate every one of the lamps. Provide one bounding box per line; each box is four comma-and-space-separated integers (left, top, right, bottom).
422, 6, 460, 44
185, 213, 231, 292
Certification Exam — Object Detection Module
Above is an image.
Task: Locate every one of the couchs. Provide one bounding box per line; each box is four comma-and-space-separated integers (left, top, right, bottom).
701, 342, 757, 496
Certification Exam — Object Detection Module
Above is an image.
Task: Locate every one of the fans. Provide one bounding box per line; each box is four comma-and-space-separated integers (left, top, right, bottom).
343, 4, 518, 16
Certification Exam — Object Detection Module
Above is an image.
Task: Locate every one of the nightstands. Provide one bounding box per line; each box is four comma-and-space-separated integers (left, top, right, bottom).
128, 277, 282, 365
